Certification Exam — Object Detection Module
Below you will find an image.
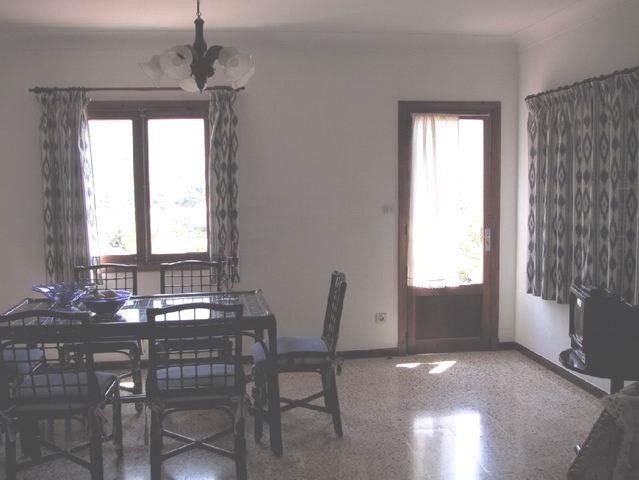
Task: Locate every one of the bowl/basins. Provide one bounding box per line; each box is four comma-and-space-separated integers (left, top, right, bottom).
29, 282, 97, 308
77, 288, 133, 318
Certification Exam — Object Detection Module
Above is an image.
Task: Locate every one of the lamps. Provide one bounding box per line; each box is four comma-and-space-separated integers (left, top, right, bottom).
138, 1, 256, 94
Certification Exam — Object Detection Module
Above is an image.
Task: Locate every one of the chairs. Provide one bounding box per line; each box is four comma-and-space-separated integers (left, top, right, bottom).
252, 271, 347, 456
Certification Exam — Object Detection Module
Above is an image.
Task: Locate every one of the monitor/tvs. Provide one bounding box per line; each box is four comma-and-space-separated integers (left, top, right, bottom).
569, 285, 639, 366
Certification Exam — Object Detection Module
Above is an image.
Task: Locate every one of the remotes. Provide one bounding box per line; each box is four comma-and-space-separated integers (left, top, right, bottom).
581, 284, 600, 292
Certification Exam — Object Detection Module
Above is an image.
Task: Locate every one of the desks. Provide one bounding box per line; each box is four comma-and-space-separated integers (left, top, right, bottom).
560, 349, 639, 395
